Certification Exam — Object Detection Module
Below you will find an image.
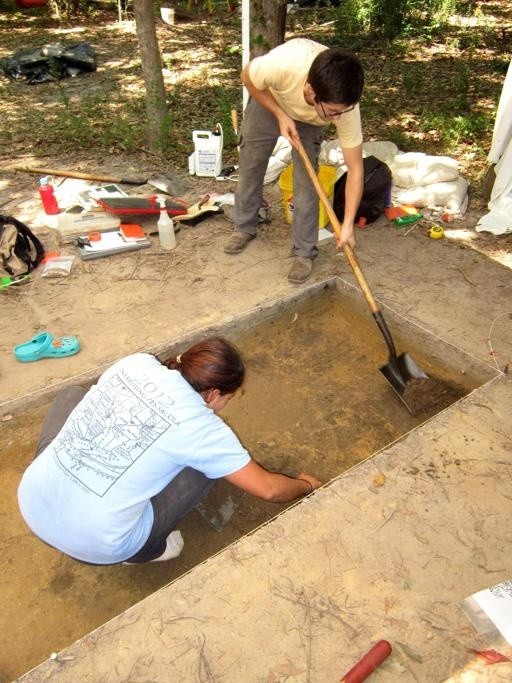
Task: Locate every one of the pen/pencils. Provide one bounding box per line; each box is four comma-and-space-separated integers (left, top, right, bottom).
118, 233, 127, 243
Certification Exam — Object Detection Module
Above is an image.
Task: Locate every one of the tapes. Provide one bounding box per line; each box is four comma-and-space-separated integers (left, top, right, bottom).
430, 226, 444, 239
88, 231, 102, 241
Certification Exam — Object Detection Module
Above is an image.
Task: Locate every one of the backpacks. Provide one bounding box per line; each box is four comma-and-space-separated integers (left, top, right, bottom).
0, 215, 45, 277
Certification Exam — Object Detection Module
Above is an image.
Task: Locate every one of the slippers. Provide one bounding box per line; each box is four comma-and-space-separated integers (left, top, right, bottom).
13, 331, 80, 362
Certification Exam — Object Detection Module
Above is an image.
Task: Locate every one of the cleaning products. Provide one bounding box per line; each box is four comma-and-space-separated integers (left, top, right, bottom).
395, 213, 423, 225
154, 196, 176, 250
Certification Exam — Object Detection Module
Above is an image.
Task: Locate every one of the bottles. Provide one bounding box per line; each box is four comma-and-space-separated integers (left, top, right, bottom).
38, 173, 61, 215
151, 195, 178, 250
422, 207, 454, 223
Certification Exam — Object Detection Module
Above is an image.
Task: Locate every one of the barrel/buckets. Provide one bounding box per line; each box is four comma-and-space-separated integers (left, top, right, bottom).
159, 7, 176, 25
278, 160, 338, 230
193, 126, 223, 178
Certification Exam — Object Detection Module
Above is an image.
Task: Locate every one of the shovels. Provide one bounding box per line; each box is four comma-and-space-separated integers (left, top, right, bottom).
289, 126, 449, 418
15, 165, 189, 197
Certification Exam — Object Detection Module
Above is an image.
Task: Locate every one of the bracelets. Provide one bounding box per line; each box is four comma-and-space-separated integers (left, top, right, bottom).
299, 479, 312, 492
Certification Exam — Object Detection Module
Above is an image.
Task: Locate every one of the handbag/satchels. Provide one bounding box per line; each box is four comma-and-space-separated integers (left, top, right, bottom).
332, 154, 392, 228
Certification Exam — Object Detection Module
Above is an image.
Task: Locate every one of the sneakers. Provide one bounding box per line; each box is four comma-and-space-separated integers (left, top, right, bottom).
287, 256, 313, 284
220, 204, 270, 224
122, 530, 184, 565
223, 231, 256, 253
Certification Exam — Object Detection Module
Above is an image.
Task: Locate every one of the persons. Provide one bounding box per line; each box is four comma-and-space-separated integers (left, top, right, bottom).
223, 36, 365, 284
17, 335, 323, 567
